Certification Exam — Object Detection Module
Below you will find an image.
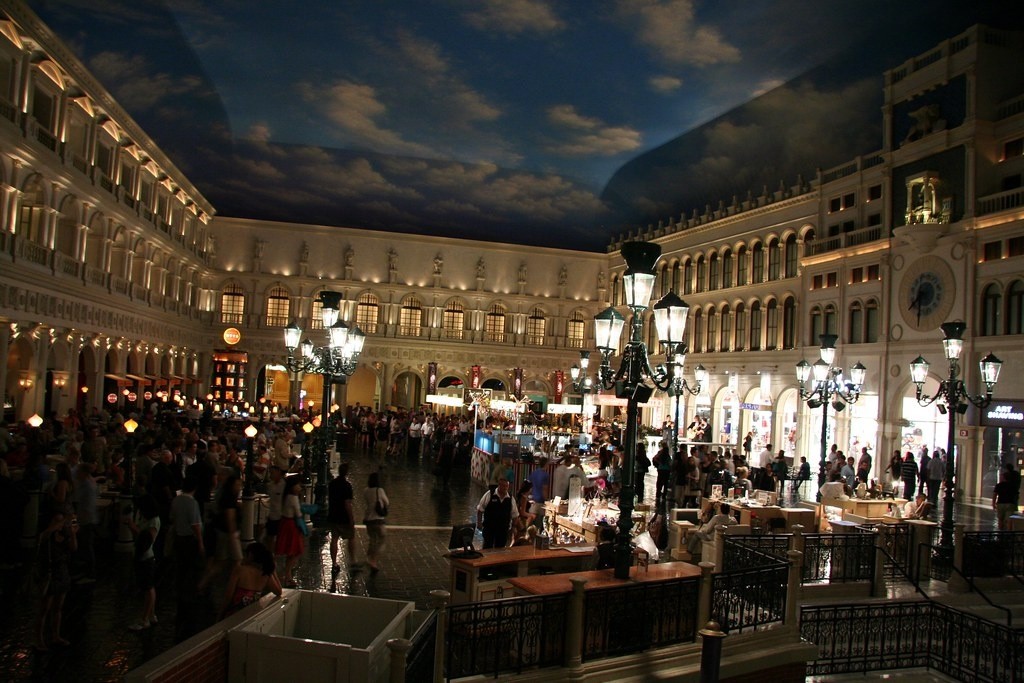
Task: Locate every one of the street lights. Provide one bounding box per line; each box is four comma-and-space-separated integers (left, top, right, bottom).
650, 345, 707, 488
908, 321, 1005, 566
792, 330, 867, 502
590, 239, 691, 580
570, 348, 592, 434
282, 290, 370, 527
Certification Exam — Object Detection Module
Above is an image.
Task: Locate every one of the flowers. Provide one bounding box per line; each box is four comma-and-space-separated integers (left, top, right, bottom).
592, 478, 607, 499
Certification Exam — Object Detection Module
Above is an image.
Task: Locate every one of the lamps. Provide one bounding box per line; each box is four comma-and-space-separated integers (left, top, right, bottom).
54, 378, 65, 389
18, 379, 34, 390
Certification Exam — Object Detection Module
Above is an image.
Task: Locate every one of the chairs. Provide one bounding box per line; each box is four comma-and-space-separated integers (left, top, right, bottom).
700, 524, 752, 571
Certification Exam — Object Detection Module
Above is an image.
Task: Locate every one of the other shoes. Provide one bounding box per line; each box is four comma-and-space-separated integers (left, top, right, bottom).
285, 579, 301, 588
36, 643, 52, 651
129, 614, 158, 630
370, 560, 379, 571
331, 564, 340, 573
52, 637, 69, 646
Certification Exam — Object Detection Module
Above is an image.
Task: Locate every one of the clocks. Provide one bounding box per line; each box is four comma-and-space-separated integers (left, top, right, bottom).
898, 255, 956, 333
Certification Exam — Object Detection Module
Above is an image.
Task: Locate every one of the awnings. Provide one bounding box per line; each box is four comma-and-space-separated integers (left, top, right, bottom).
144, 375, 167, 386
104, 374, 133, 386
161, 376, 202, 385
126, 375, 151, 386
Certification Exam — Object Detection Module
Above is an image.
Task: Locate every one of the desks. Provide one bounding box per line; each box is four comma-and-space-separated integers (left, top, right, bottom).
441, 541, 649, 631
506, 560, 701, 670
785, 477, 797, 494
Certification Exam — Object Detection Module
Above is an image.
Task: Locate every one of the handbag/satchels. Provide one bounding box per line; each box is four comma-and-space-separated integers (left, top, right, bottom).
375, 488, 388, 517
690, 478, 701, 491
18, 575, 49, 602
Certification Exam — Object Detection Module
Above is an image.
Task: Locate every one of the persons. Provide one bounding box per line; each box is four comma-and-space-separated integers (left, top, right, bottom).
652, 414, 809, 555
598, 271, 606, 288
302, 240, 310, 262
477, 258, 487, 277
0, 407, 391, 653
886, 494, 927, 519
992, 463, 1021, 531
345, 245, 354, 265
254, 234, 263, 258
207, 235, 217, 254
434, 253, 443, 275
349, 400, 474, 466
388, 248, 397, 269
590, 429, 650, 502
559, 264, 568, 284
518, 262, 528, 281
885, 448, 947, 504
476, 437, 617, 570
824, 444, 871, 495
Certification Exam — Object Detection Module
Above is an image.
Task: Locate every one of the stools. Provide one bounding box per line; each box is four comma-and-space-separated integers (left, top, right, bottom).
803, 477, 813, 494
